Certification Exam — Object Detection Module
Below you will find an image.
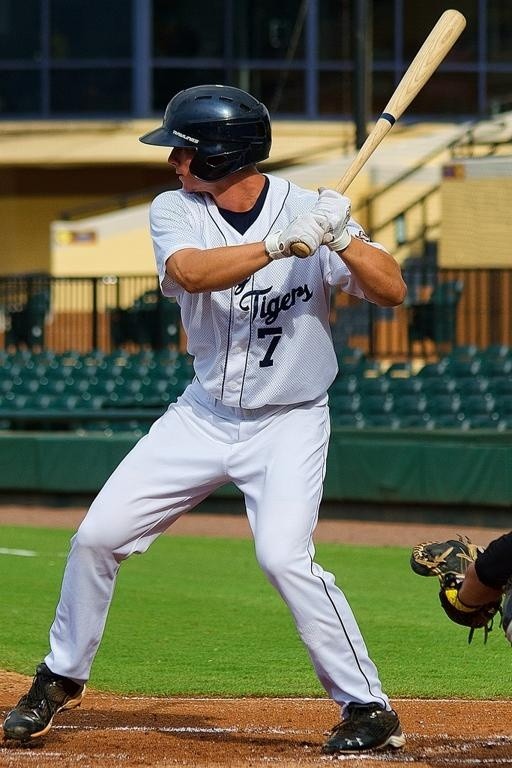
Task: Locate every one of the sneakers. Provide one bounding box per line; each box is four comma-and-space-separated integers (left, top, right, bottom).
323, 701, 406, 753
4, 662, 86, 739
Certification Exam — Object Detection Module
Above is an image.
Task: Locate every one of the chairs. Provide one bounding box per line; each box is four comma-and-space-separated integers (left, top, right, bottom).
0, 278, 512, 446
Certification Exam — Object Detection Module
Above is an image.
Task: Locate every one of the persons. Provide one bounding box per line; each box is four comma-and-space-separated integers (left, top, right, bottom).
411, 528, 511, 646
3, 84, 409, 755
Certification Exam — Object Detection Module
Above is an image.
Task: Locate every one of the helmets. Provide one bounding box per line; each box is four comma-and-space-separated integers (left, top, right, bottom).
139, 85, 272, 183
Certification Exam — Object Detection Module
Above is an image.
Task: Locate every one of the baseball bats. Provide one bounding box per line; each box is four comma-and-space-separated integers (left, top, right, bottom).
290, 9, 466, 256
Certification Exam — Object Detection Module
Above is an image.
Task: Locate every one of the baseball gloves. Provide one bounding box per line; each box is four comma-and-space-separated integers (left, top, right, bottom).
410, 535, 502, 644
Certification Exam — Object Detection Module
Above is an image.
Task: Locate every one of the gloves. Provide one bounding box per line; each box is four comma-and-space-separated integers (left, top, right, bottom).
266, 187, 351, 260
